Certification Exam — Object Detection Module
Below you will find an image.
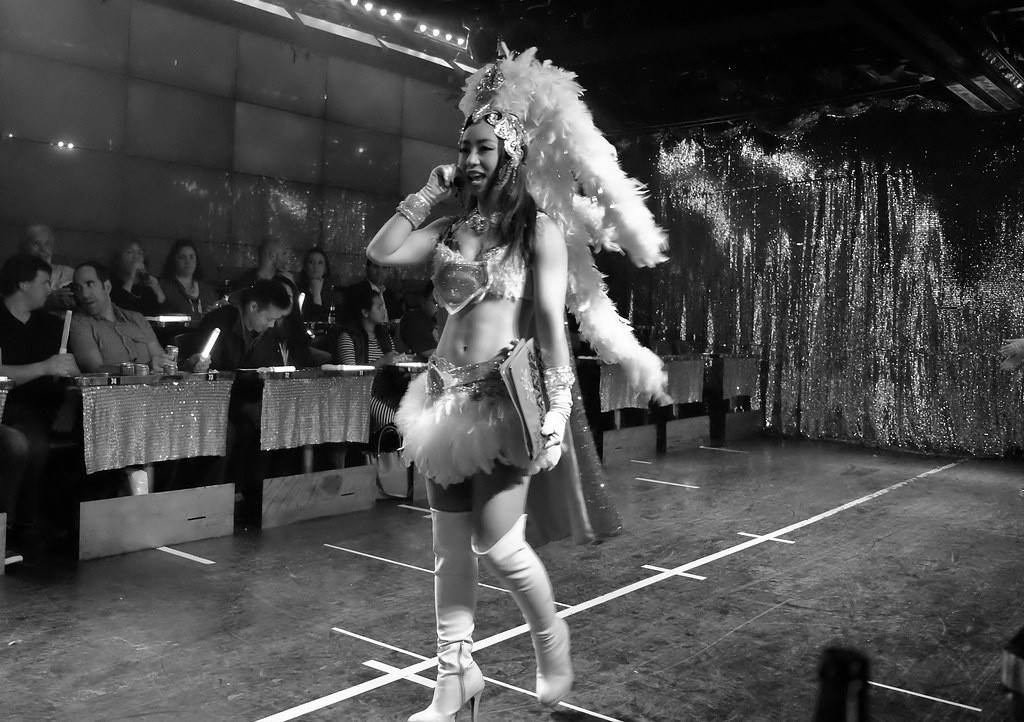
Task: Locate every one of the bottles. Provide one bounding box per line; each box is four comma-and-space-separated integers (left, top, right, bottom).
328, 303, 335, 324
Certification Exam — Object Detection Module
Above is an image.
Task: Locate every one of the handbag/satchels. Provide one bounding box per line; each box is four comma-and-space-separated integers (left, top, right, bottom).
361, 426, 413, 500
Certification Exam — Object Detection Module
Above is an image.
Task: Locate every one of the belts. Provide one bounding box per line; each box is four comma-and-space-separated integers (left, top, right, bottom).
424, 356, 507, 398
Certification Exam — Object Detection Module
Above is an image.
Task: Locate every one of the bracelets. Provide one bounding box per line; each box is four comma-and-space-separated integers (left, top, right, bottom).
397, 194, 432, 227
542, 365, 575, 388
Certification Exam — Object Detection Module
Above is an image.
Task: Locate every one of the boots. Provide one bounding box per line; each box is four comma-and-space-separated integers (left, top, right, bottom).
471, 513, 574, 708
406, 506, 486, 722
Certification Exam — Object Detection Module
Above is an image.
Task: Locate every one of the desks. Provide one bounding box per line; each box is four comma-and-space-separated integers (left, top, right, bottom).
0, 344, 763, 565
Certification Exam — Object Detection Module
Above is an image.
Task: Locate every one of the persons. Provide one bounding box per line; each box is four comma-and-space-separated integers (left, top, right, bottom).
0, 224, 436, 571
365, 39, 670, 722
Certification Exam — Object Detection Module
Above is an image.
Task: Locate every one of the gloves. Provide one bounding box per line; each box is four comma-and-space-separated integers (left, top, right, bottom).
540, 366, 575, 449
395, 163, 463, 230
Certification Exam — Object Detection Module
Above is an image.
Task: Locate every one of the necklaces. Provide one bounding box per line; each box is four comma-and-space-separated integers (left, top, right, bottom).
463, 206, 507, 233
132, 286, 143, 298
